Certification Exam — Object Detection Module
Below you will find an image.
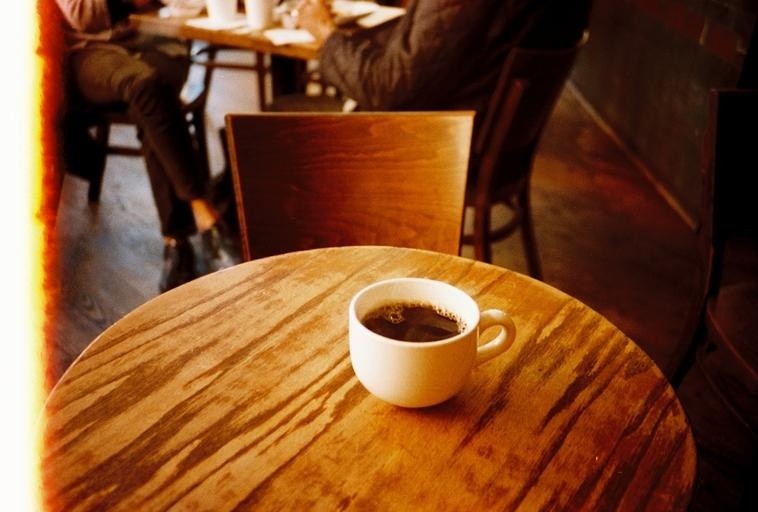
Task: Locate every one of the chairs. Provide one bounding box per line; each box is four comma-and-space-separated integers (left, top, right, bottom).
223, 109, 478, 259
460, 12, 586, 282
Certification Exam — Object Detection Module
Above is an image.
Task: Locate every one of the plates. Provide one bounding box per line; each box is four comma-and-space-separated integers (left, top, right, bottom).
275, 0, 379, 29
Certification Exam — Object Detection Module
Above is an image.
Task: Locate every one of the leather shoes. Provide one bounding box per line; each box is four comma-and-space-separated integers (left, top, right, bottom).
157, 236, 199, 294
200, 212, 243, 273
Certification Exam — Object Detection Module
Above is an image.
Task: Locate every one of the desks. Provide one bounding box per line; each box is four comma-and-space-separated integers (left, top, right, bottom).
123, 10, 324, 114
37, 243, 698, 512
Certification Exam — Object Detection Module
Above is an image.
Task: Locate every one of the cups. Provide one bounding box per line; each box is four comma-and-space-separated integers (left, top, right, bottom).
347, 276, 516, 408
205, 0, 273, 30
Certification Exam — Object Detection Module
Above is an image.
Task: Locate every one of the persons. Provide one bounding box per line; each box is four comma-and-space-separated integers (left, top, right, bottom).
211, 1, 591, 261
60, 0, 224, 295
